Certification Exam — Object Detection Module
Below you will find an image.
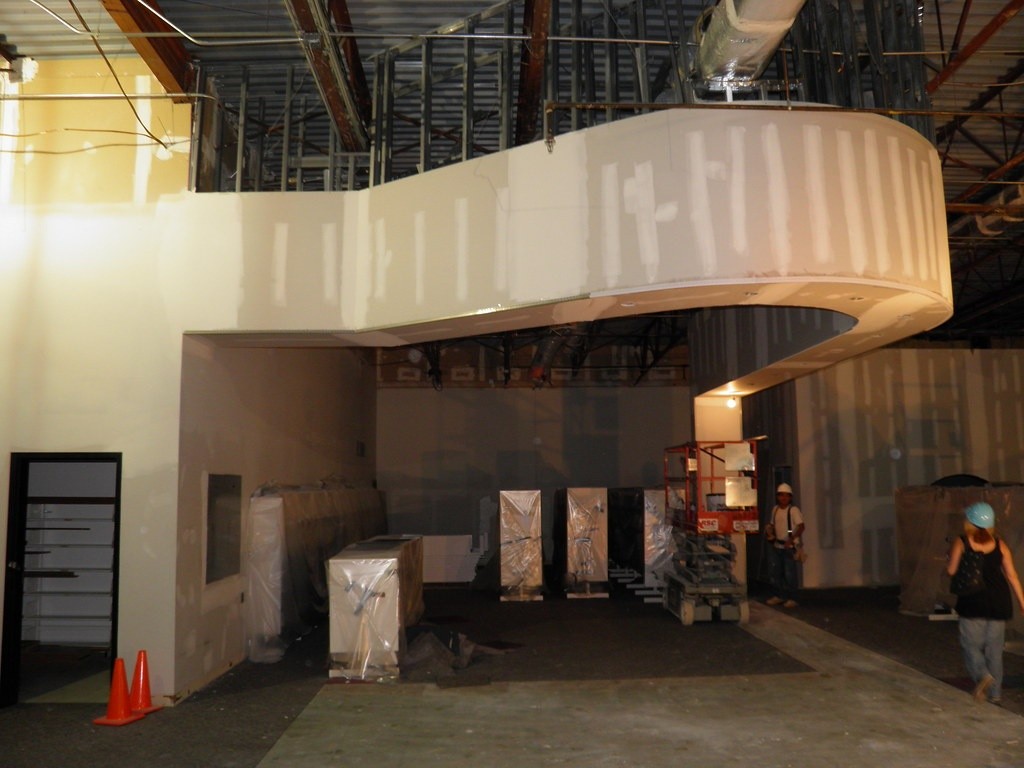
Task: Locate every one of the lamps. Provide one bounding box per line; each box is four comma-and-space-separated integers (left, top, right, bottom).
9, 57, 40, 83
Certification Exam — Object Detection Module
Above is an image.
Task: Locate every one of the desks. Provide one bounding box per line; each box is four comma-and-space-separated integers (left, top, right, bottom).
325, 535, 425, 663
242, 488, 388, 636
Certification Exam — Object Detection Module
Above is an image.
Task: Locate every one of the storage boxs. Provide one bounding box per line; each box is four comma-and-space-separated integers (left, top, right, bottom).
643, 490, 748, 589
499, 490, 544, 588
895, 484, 1024, 614
567, 487, 609, 583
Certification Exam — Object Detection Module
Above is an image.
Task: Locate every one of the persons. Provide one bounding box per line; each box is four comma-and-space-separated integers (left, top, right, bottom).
764, 483, 806, 608
947, 502, 1024, 704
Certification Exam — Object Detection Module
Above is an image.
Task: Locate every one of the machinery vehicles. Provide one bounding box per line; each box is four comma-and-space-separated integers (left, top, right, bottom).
662, 435, 760, 627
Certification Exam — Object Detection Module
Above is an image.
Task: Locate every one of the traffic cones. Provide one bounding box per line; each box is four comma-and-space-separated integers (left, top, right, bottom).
128, 650, 163, 716
93, 658, 143, 726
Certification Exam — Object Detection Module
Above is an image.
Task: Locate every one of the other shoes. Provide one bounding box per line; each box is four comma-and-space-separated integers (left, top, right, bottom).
986, 692, 1004, 702
766, 597, 780, 604
784, 600, 796, 607
975, 675, 995, 698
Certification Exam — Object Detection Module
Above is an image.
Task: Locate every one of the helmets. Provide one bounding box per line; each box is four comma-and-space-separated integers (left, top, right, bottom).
963, 502, 996, 529
777, 482, 793, 493
